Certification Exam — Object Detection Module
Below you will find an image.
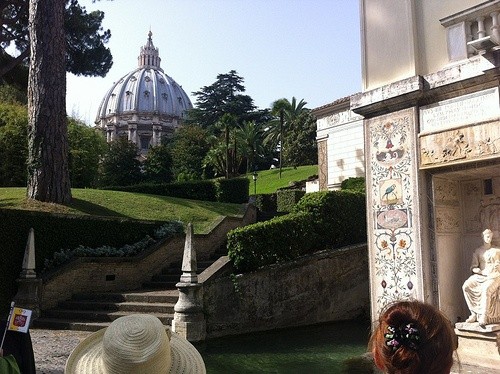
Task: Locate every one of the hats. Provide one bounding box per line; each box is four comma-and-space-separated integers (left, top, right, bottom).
64, 314, 206, 373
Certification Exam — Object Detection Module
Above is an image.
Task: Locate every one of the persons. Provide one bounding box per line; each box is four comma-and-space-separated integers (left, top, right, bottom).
369, 300, 458, 374
463, 229, 499, 324
